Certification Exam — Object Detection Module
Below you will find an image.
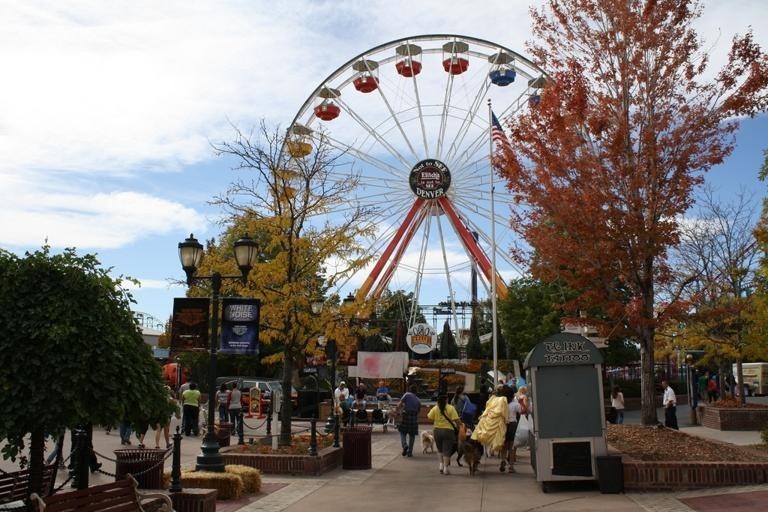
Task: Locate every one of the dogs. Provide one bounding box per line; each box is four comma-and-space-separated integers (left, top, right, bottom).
457, 438, 483, 475
420, 430, 435, 455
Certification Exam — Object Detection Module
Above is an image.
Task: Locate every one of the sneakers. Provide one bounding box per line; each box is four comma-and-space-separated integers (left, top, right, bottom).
499, 460, 516, 473
45, 459, 102, 473
121, 438, 172, 450
401, 444, 413, 457
439, 466, 450, 474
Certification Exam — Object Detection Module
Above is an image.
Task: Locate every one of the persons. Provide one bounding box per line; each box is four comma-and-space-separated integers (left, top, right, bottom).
494, 387, 525, 472
339, 395, 349, 427
707, 376, 718, 403
68, 422, 102, 472
611, 385, 625, 424
120, 424, 131, 445
215, 383, 231, 421
661, 381, 678, 430
497, 384, 529, 461
726, 374, 737, 395
398, 385, 421, 457
46, 427, 64, 467
451, 385, 477, 425
178, 377, 192, 433
135, 424, 148, 448
155, 386, 172, 449
355, 383, 364, 408
182, 384, 201, 436
376, 381, 388, 409
335, 381, 349, 406
227, 382, 243, 435
427, 392, 461, 472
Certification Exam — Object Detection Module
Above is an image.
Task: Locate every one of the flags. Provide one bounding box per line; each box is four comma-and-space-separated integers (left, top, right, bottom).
492, 112, 513, 160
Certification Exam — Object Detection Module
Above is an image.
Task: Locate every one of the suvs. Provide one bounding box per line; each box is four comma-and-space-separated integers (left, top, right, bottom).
215, 378, 283, 410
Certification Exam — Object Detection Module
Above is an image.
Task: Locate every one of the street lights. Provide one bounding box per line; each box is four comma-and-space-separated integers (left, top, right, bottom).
686, 352, 697, 410
175, 226, 263, 473
308, 288, 361, 433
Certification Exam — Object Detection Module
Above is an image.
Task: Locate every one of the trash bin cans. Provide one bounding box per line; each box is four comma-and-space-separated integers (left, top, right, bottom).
112, 449, 164, 489
298, 390, 314, 418
596, 456, 623, 494
203, 423, 232, 448
340, 426, 373, 470
605, 407, 617, 424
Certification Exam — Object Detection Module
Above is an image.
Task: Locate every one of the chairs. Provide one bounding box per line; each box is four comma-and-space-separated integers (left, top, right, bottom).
341, 409, 395, 433
